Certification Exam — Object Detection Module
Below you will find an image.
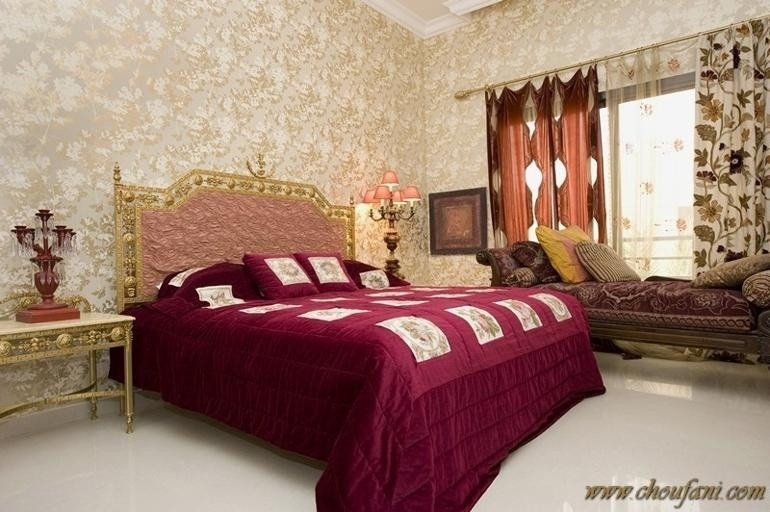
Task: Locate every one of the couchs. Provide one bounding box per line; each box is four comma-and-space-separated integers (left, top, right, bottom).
476, 247, 769, 365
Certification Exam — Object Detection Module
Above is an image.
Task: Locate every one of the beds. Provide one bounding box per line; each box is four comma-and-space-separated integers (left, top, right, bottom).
113, 150, 606, 511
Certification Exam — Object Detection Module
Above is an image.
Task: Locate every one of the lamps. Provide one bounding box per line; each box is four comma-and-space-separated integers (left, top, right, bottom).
11, 209, 80, 323
362, 170, 421, 279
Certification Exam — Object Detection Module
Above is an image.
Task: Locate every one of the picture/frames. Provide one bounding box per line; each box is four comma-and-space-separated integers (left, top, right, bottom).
428, 187, 487, 255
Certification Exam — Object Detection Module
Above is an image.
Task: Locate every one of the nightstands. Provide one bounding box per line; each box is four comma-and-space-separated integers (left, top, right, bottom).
0, 293, 136, 434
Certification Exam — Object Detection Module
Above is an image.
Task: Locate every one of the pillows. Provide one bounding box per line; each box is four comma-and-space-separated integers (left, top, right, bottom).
242, 252, 320, 300
689, 253, 770, 288
510, 241, 560, 283
293, 251, 359, 293
575, 240, 641, 283
535, 224, 595, 283
